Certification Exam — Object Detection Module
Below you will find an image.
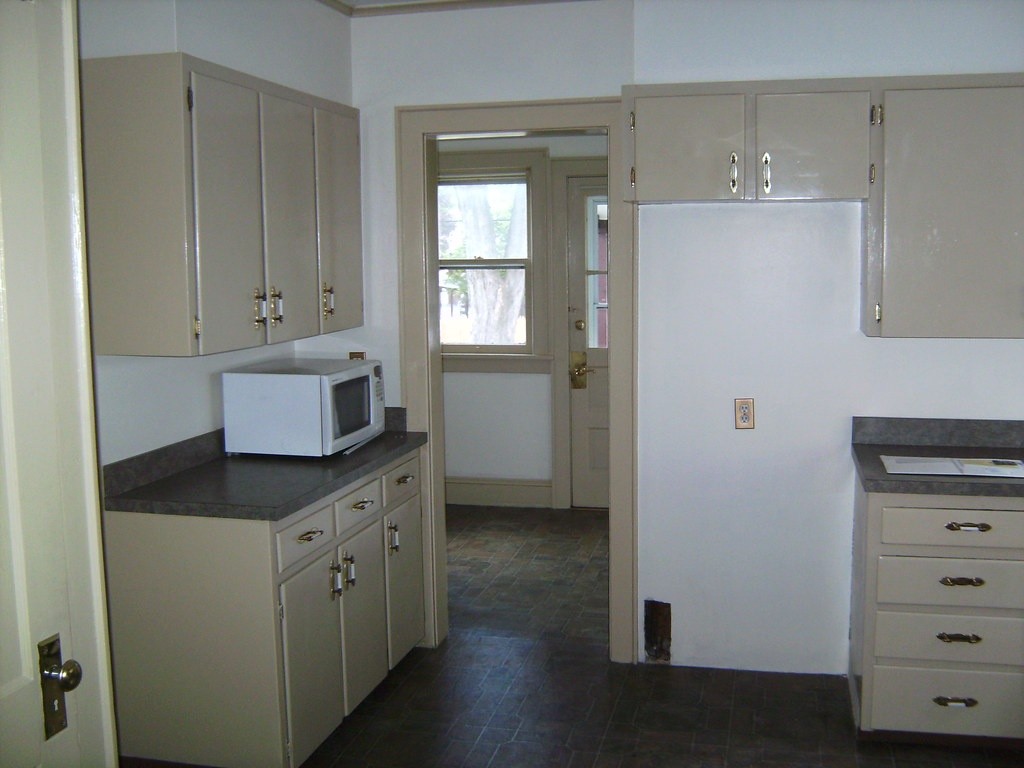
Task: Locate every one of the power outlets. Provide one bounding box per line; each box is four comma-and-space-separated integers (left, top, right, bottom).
349, 352, 366, 360
734, 398, 755, 429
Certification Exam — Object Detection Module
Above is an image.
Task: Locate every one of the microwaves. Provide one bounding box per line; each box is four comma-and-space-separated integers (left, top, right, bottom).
221, 357, 386, 459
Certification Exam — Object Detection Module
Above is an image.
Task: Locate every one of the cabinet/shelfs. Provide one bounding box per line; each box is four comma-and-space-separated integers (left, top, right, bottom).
104, 433, 428, 768
621, 72, 1024, 339
80, 52, 365, 358
847, 469, 1024, 739
